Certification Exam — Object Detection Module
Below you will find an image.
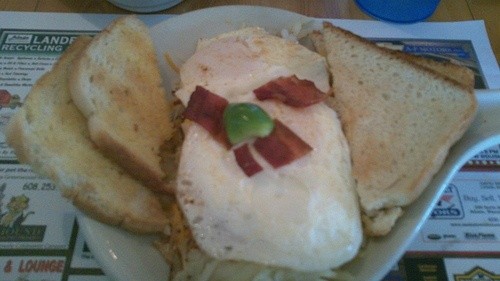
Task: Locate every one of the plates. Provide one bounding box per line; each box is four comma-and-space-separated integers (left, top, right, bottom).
78, 5, 500, 281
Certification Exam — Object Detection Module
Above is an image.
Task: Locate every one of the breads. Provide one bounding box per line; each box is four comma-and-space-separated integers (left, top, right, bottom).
7, 36, 168, 234
310, 20, 479, 236
70, 13, 181, 192
